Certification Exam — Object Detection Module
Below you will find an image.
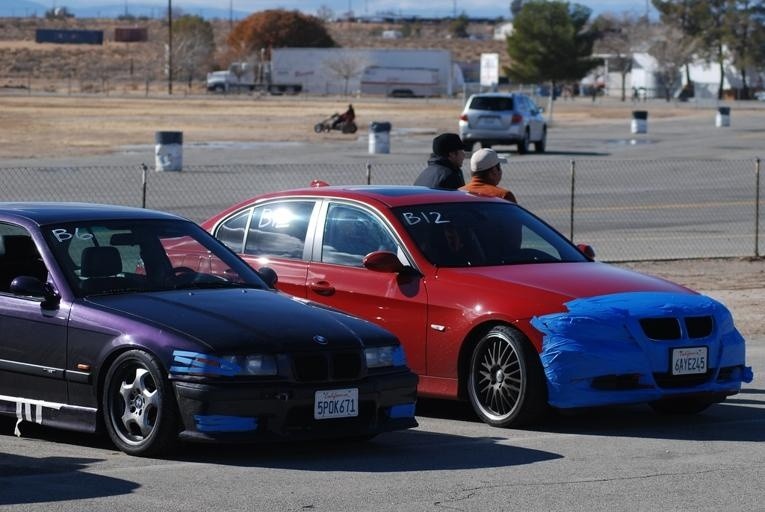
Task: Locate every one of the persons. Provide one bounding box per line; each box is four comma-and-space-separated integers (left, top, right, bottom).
458, 149, 522, 253
332, 102, 354, 131
411, 132, 464, 195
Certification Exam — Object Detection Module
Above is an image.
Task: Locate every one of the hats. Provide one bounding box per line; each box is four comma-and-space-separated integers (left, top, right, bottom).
432, 133, 465, 154
470, 148, 509, 172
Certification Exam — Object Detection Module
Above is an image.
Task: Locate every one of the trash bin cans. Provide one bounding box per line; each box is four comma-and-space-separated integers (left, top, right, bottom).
369, 122, 390, 153
154, 131, 183, 174
717, 107, 730, 127
632, 110, 647, 133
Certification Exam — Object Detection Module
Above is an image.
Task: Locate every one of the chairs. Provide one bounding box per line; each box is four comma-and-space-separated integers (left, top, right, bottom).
0, 234, 136, 297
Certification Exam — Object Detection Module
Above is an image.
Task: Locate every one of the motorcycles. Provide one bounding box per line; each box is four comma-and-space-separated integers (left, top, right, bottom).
316, 116, 356, 133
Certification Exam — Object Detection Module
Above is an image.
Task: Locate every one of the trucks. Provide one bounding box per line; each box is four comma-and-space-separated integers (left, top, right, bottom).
208, 49, 453, 96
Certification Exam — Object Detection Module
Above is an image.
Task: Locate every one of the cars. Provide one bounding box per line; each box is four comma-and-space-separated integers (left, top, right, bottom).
1, 202, 420, 456
134, 181, 752, 429
458, 93, 547, 154
